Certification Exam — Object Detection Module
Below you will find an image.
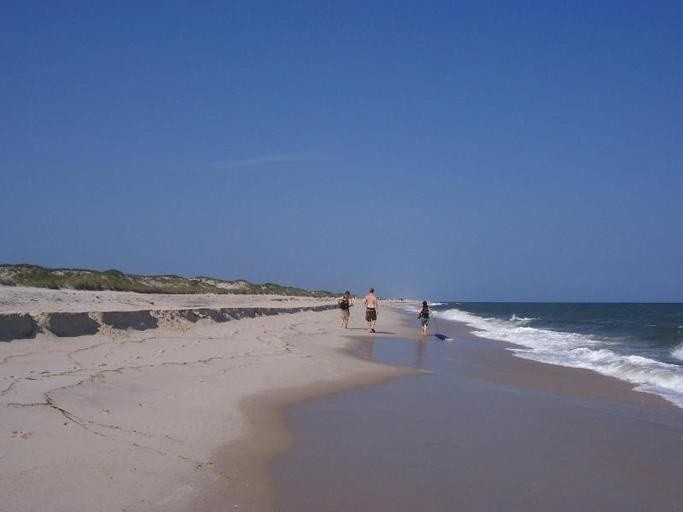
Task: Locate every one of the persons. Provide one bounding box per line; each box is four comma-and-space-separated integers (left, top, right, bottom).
338, 291, 352, 329
417, 301, 435, 336
363, 288, 379, 333
